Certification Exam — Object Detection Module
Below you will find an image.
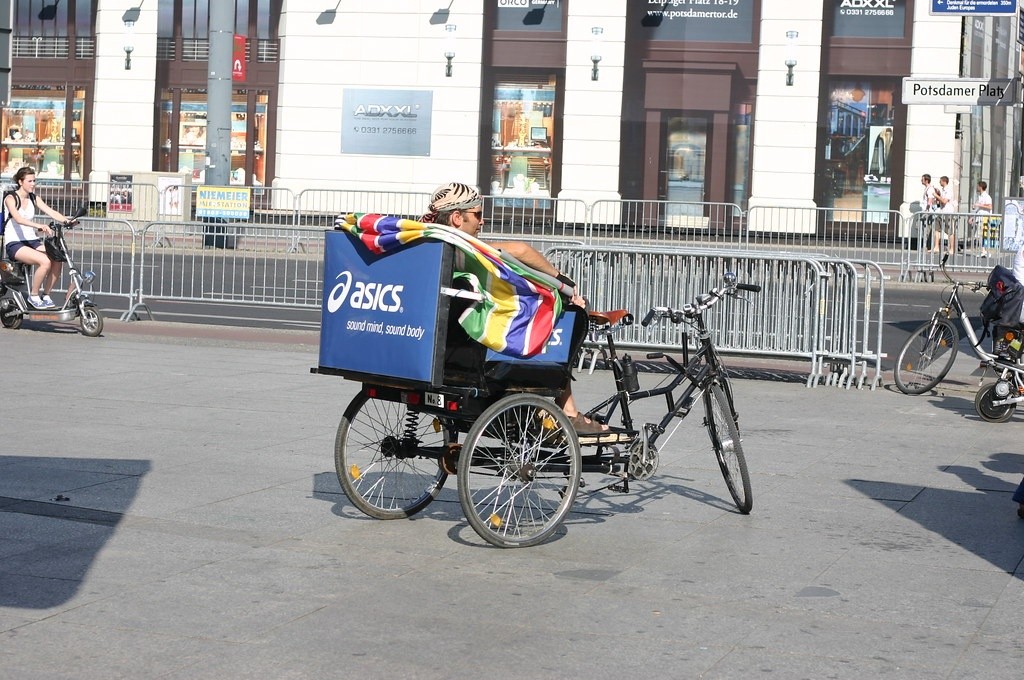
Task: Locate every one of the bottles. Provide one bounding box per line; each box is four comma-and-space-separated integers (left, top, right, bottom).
516, 175, 527, 193
622, 353, 640, 392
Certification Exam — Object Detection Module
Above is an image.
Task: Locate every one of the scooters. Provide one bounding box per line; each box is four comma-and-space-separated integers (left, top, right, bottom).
0, 208, 104, 337
894, 252, 1024, 423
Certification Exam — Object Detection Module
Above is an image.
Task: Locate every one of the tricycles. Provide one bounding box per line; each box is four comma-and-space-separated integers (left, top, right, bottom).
310, 230, 761, 549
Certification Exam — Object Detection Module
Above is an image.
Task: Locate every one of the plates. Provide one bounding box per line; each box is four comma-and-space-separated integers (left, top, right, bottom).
491, 188, 501, 194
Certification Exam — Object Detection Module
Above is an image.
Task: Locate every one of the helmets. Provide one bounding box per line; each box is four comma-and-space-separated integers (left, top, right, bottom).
44, 236, 67, 263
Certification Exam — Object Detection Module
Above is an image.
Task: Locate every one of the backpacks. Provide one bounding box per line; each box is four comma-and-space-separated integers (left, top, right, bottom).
926, 186, 942, 208
1, 191, 37, 234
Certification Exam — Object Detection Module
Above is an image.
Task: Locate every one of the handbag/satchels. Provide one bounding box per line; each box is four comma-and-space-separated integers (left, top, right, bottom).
980, 266, 1024, 326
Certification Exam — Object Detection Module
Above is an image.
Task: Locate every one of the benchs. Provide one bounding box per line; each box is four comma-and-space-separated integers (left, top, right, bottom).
320, 227, 581, 392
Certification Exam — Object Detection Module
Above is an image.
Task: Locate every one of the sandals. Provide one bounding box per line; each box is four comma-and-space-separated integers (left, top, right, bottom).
562, 412, 612, 437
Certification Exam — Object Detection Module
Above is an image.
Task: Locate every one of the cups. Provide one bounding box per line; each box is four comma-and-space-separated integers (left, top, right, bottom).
530, 182, 539, 196
887, 177, 891, 184
492, 181, 500, 190
881, 177, 886, 184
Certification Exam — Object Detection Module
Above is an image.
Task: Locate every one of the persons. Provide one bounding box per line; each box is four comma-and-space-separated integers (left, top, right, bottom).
921, 174, 956, 254
416, 182, 609, 435
3, 167, 76, 311
967, 182, 993, 258
1012, 238, 1024, 383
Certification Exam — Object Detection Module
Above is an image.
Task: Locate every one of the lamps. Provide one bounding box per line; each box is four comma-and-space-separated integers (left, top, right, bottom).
125, 21, 134, 70
445, 24, 456, 78
785, 30, 800, 86
590, 26, 604, 80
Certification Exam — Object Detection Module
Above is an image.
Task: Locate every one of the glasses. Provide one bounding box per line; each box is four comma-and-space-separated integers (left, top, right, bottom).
458, 210, 482, 220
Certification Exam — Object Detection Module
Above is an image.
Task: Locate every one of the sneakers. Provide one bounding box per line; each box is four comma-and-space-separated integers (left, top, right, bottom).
28, 295, 46, 310
40, 293, 55, 308
